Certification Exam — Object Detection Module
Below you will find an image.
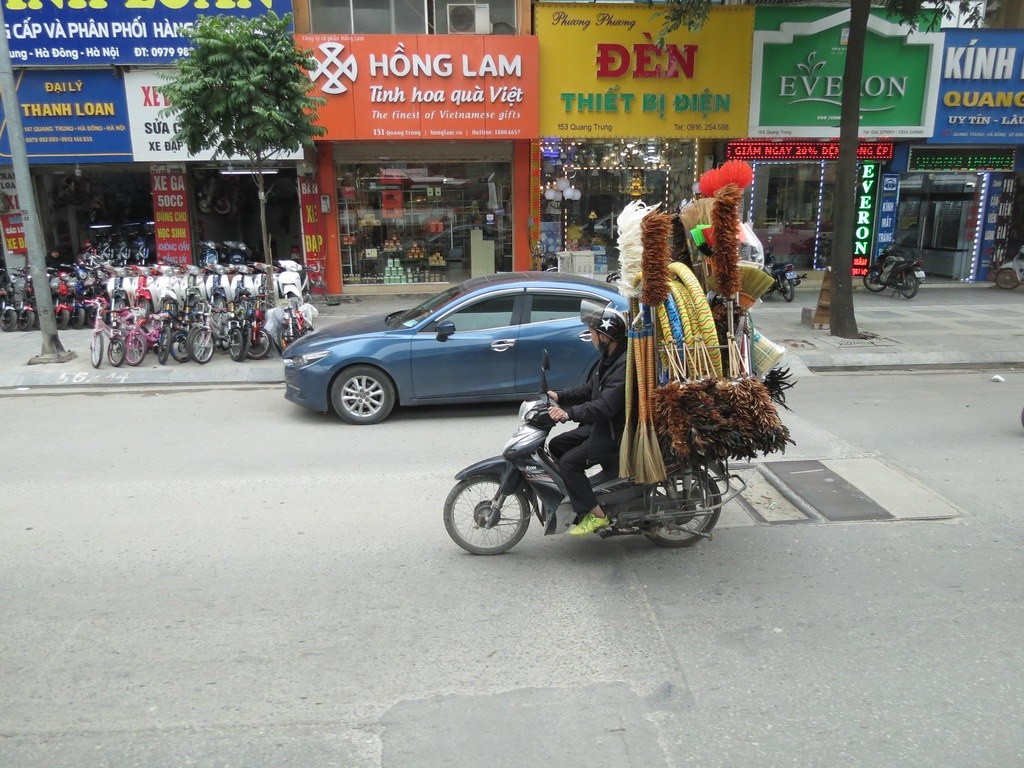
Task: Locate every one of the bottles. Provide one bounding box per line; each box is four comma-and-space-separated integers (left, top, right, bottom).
343, 272, 384, 284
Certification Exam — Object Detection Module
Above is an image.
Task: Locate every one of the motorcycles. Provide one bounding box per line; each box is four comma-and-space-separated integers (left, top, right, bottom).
863, 240, 926, 299
765, 254, 807, 304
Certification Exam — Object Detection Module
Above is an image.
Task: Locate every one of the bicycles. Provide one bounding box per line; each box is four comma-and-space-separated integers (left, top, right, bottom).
82, 288, 313, 368
198, 191, 233, 216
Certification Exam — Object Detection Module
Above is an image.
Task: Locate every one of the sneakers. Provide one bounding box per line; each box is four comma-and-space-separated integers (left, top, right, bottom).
567, 513, 612, 537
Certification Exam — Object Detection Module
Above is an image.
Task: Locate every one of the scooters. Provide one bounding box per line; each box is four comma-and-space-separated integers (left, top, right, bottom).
46, 265, 109, 330
84, 229, 154, 265
193, 241, 226, 267
995, 245, 1024, 289
107, 265, 312, 307
443, 348, 748, 556
225, 242, 251, 265
0, 266, 41, 331
86, 180, 105, 226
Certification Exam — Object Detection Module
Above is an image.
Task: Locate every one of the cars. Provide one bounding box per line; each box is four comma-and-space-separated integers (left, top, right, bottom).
402, 223, 513, 263
281, 271, 629, 425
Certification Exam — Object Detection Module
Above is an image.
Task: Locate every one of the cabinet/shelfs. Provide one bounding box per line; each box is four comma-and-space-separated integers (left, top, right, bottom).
338, 177, 512, 283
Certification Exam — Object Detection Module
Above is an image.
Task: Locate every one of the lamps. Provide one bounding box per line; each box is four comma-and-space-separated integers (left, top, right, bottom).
588, 211, 598, 223
74, 164, 81, 176
545, 201, 562, 222
227, 163, 234, 172
544, 175, 581, 211
618, 170, 656, 195
220, 169, 280, 175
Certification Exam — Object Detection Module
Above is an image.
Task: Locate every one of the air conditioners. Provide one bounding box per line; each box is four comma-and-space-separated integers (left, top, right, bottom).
447, 3, 494, 35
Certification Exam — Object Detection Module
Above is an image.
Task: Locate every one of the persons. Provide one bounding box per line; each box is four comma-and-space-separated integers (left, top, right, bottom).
544, 308, 639, 536
46, 247, 69, 276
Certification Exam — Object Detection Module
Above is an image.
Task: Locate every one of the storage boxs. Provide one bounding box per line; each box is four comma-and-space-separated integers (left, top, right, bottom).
591, 245, 607, 256
421, 220, 436, 234
594, 264, 609, 274
434, 221, 444, 234
370, 220, 381, 226
595, 255, 608, 264
357, 220, 369, 226
363, 213, 375, 220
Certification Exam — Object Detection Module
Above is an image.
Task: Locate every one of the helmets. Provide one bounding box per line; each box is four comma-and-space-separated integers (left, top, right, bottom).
587, 308, 627, 342
